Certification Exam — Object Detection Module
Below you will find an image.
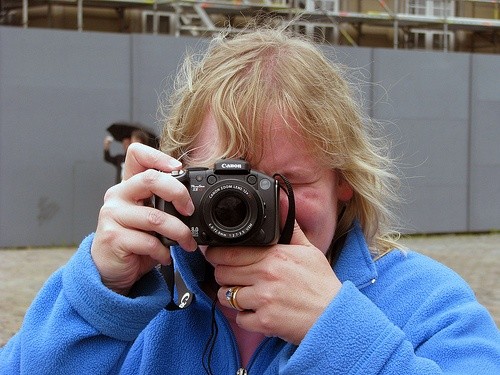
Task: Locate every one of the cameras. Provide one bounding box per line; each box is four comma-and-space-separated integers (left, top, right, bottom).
155, 159, 281, 248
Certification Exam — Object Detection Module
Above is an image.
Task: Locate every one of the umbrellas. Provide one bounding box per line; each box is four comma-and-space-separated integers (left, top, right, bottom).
105, 119, 160, 150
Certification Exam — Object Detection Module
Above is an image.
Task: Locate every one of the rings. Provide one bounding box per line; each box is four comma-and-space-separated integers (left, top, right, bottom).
226, 286, 243, 313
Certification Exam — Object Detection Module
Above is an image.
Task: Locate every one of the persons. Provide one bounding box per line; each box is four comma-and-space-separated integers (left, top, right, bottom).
0, 30, 500, 375
102, 137, 131, 186
